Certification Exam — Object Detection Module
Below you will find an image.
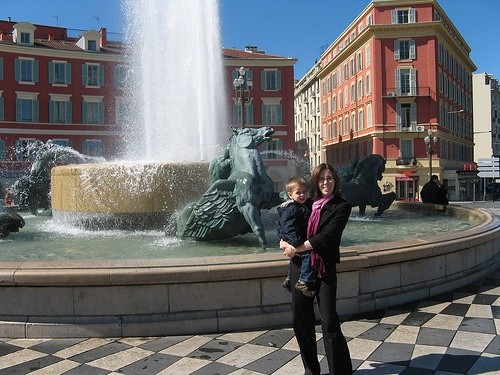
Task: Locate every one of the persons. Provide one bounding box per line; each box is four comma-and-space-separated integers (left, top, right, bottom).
420, 175, 439, 203
493, 184, 500, 205
208, 143, 232, 188
279, 163, 352, 375
437, 183, 448, 205
277, 177, 317, 297
4, 188, 12, 206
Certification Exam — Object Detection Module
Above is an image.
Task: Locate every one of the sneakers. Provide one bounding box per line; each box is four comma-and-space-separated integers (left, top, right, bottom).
295, 281, 313, 296
283, 278, 292, 293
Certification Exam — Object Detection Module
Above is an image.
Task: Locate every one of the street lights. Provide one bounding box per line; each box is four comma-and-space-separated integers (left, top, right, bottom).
423, 128, 438, 182
233, 66, 254, 129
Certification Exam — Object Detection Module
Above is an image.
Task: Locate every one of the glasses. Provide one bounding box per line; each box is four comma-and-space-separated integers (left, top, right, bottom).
317, 176, 335, 183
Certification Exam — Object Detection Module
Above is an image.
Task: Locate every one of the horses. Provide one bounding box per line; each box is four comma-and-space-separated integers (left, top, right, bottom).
339, 154, 397, 218
167, 126, 290, 251
